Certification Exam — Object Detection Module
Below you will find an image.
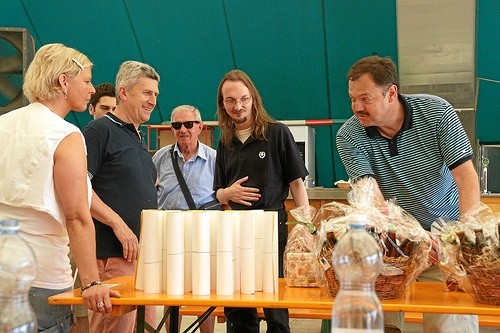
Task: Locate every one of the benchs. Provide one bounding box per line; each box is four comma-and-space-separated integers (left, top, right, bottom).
179, 306, 500, 333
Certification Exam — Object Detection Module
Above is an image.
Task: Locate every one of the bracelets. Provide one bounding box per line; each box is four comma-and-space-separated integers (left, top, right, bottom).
80, 281, 102, 293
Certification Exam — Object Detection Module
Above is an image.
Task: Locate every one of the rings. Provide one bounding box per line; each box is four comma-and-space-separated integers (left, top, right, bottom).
96, 302, 104, 308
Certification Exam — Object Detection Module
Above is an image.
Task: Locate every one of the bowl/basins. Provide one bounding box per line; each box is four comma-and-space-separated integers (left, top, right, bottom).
337, 183, 350, 188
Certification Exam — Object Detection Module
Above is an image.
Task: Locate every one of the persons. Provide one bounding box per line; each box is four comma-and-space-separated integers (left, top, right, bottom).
336, 55, 479, 333
152, 105, 217, 333
213, 69, 310, 333
83, 59, 158, 333
69, 84, 117, 332
0, 43, 121, 333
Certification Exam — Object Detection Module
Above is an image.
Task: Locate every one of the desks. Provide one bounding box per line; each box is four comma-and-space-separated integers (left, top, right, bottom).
48, 276, 500, 333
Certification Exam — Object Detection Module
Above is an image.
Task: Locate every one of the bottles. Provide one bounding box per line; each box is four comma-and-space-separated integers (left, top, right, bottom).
331, 213, 383, 333
0, 218, 39, 333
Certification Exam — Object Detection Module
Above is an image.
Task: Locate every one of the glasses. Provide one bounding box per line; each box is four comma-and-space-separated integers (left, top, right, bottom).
171, 121, 199, 130
222, 95, 254, 104
